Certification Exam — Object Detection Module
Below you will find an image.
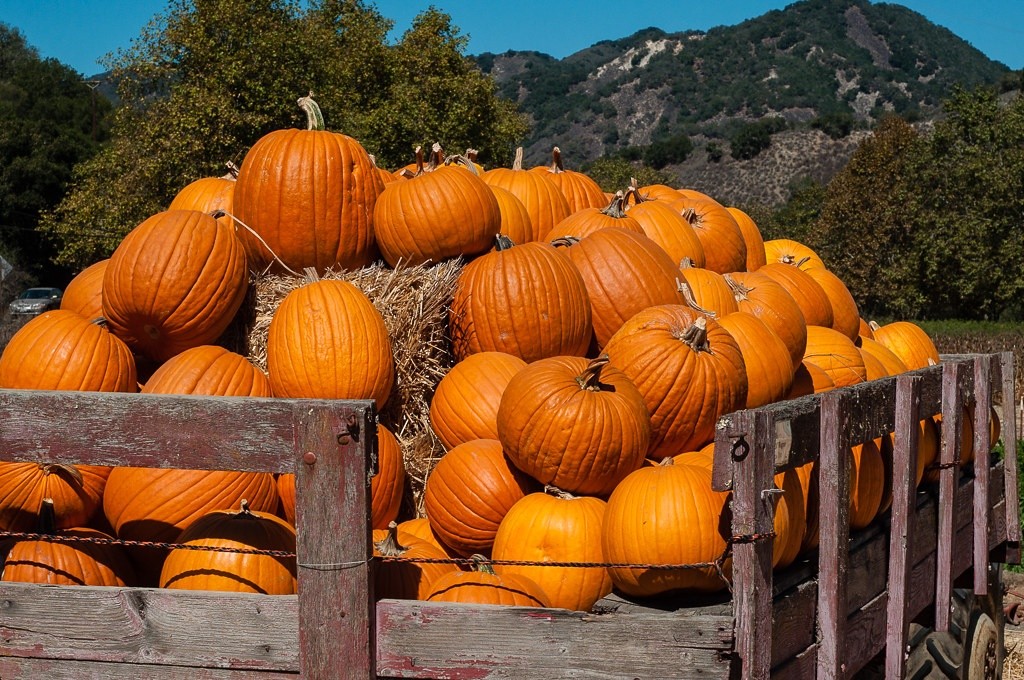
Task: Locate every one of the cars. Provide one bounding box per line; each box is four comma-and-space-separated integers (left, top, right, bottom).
8, 287, 63, 320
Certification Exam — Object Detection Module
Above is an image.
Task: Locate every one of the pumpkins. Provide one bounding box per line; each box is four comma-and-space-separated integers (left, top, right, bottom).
0, 98, 1001, 608
